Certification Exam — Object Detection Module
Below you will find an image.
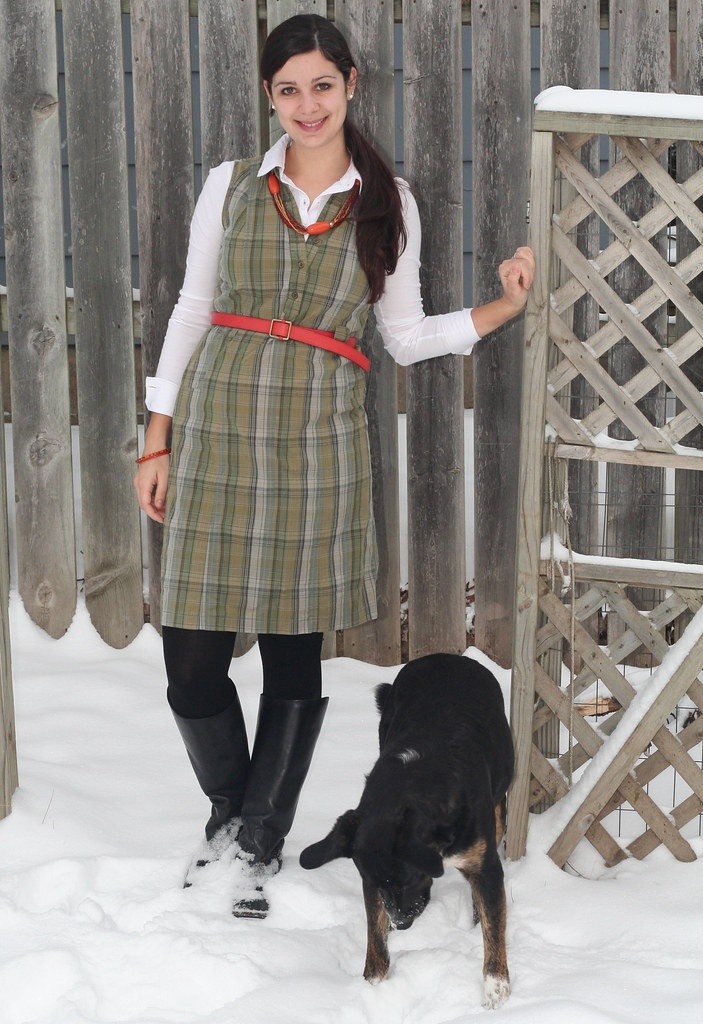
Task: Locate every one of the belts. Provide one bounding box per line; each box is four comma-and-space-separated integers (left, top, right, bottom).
212, 311, 370, 372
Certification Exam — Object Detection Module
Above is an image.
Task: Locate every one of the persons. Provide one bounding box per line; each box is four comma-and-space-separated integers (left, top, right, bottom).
134, 14, 535, 920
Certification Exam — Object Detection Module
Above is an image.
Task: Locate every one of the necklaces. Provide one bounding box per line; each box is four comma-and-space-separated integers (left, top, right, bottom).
266, 172, 361, 235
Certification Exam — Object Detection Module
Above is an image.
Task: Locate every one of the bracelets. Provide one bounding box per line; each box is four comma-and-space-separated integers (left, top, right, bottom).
136, 449, 169, 464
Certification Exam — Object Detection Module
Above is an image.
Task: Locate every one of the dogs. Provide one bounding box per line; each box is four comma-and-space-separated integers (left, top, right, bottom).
298, 652, 517, 1011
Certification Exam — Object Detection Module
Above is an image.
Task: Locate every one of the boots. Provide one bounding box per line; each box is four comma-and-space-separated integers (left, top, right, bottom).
167, 677, 251, 888
233, 694, 329, 919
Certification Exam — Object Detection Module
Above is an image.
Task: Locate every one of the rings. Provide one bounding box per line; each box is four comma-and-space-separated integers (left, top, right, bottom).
514, 253, 517, 259
